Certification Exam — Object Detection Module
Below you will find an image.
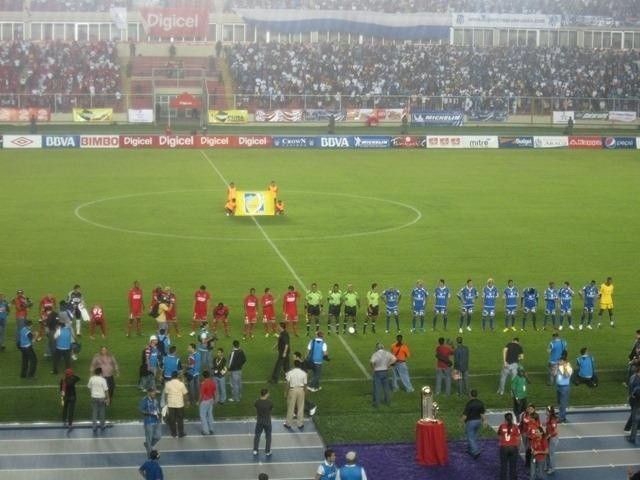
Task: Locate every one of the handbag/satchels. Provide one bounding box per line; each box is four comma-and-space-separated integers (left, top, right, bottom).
435, 352, 452, 368
304, 356, 314, 368
70, 342, 81, 352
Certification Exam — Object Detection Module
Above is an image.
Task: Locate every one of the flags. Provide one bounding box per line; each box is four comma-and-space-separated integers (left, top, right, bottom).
235, 190, 278, 216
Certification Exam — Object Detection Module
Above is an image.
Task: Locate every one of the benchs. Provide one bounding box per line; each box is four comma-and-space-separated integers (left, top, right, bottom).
129, 54, 228, 111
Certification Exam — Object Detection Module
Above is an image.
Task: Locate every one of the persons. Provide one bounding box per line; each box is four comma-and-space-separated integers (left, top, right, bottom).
269, 181, 278, 192
228, 183, 236, 199
224, 199, 237, 216
275, 200, 284, 215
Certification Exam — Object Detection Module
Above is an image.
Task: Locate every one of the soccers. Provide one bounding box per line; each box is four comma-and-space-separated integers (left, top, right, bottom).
348, 326, 355, 334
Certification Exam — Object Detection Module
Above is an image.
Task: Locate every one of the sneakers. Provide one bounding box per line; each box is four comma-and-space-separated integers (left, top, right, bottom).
459, 321, 616, 334
176, 332, 279, 339
172, 386, 323, 456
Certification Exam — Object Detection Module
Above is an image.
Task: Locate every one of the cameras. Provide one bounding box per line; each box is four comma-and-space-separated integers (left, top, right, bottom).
323, 356, 330, 361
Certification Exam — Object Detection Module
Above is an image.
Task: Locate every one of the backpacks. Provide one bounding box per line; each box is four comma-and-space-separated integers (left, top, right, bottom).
149, 302, 161, 318
157, 336, 166, 352
